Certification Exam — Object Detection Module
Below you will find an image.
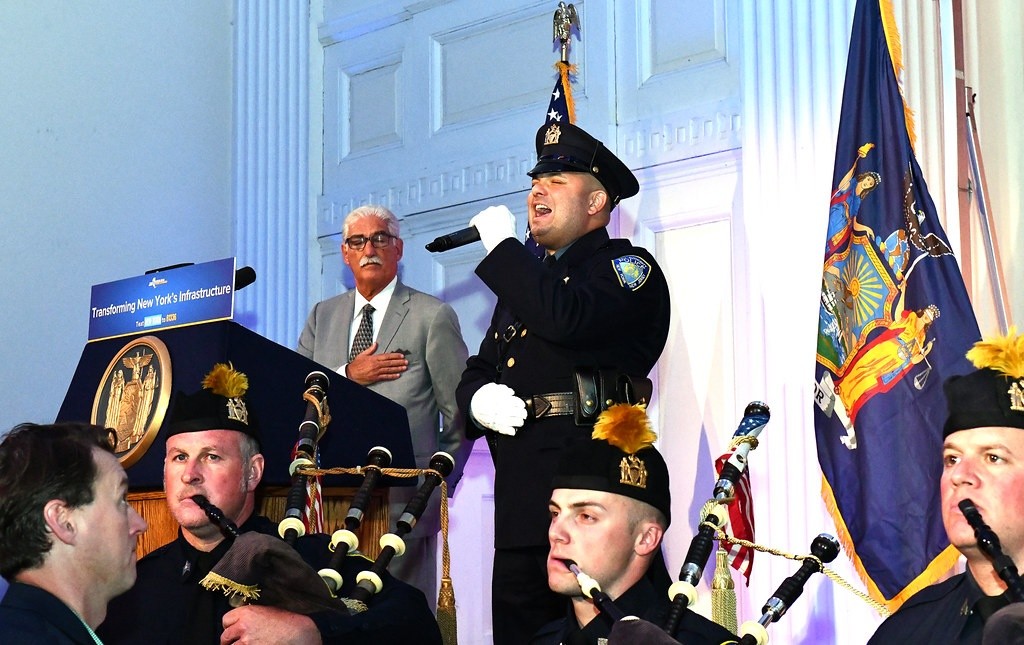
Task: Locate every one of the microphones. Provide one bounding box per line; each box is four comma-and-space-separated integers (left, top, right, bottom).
234, 267, 256, 291
424, 226, 481, 253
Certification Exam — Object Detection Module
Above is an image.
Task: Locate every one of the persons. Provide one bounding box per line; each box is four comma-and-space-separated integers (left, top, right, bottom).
297, 205, 477, 612
867, 367, 1024, 645
0, 425, 148, 645
106, 364, 442, 644
456, 120, 671, 645
529, 406, 742, 645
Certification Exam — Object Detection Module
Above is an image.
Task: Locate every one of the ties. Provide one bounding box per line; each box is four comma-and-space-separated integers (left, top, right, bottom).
975, 596, 1003, 625
191, 553, 214, 645
544, 256, 556, 269
349, 304, 376, 365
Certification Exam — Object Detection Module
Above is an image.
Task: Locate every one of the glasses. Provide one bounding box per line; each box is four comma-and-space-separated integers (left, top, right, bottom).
344, 231, 398, 250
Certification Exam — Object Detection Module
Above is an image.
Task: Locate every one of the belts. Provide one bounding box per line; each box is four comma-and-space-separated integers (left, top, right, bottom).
521, 391, 575, 419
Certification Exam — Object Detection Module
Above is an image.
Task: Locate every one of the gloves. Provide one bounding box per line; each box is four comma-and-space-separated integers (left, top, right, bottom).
470, 382, 528, 436
469, 205, 518, 254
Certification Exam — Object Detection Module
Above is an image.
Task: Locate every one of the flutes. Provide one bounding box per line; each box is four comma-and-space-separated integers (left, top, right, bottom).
958, 498, 1024, 603
563, 401, 841, 645
190, 371, 455, 617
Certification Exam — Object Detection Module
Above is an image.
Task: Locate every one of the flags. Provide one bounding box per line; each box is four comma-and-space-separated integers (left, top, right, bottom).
815, 0, 988, 612
526, 72, 573, 256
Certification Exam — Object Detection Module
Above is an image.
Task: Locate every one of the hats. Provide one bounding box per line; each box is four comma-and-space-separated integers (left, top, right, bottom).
551, 404, 671, 532
527, 121, 639, 204
942, 326, 1024, 441
167, 363, 263, 454
